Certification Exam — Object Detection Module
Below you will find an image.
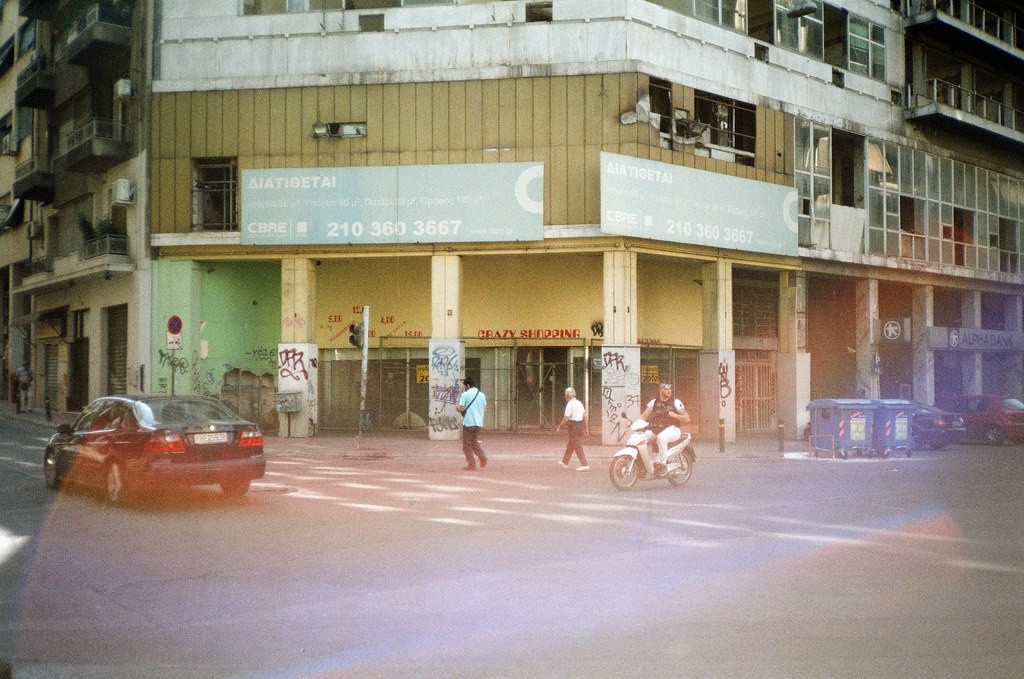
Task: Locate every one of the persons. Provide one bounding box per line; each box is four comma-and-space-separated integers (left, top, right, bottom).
556, 387, 589, 471
638, 380, 691, 477
10, 361, 35, 414
456, 376, 489, 472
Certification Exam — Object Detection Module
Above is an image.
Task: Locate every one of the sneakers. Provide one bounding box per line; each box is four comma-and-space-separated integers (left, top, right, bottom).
575, 466, 590, 471
558, 462, 568, 468
655, 466, 668, 477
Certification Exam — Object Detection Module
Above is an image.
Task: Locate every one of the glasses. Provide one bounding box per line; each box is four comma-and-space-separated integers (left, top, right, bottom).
660, 385, 673, 389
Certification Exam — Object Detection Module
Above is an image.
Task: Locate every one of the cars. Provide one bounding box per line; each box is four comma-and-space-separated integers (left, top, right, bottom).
907, 400, 965, 453
42, 393, 267, 508
936, 395, 1023, 449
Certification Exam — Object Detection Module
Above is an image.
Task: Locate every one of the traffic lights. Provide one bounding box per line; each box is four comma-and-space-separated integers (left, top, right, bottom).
348, 324, 362, 348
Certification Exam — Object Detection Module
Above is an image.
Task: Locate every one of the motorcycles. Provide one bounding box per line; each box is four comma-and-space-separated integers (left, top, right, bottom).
608, 411, 698, 489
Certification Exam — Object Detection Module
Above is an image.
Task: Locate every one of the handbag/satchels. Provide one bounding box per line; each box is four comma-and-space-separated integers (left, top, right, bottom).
462, 409, 467, 417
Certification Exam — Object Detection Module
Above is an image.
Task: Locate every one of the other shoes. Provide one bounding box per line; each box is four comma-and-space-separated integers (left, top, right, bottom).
20, 408, 31, 412
463, 465, 477, 471
481, 456, 487, 468
